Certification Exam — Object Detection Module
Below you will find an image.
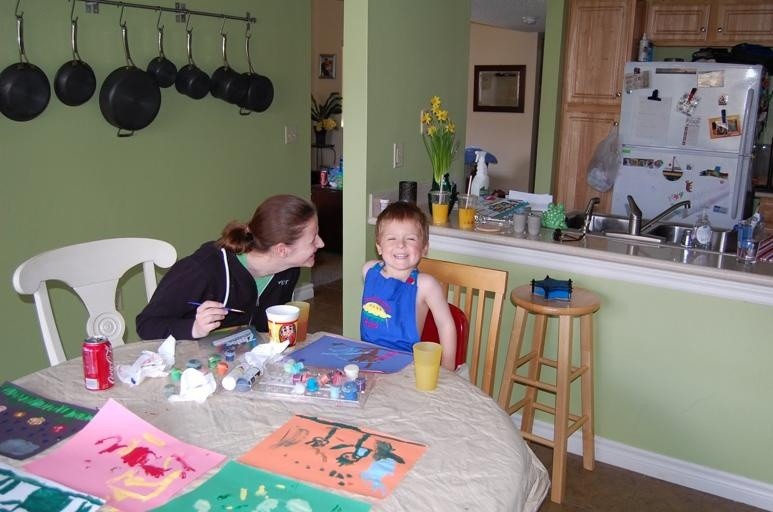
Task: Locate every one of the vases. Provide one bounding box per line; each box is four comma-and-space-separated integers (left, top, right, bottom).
314, 125, 326, 146
429, 173, 457, 215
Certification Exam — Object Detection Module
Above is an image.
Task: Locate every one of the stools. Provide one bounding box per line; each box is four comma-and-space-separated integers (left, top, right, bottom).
497, 282, 600, 504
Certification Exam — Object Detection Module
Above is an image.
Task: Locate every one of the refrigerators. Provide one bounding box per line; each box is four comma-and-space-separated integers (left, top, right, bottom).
611, 62, 762, 230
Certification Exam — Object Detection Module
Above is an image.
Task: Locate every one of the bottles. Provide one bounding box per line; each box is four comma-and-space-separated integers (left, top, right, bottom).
80, 335, 115, 392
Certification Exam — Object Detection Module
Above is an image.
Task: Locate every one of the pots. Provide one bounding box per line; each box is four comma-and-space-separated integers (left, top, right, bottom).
0, 18, 50, 123
239, 37, 273, 116
53, 21, 96, 107
98, 27, 160, 138
175, 33, 210, 100
211, 35, 246, 104
146, 30, 176, 89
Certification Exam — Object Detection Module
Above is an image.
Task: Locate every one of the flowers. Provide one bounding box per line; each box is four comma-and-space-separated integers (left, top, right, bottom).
312, 92, 342, 131
422, 97, 457, 184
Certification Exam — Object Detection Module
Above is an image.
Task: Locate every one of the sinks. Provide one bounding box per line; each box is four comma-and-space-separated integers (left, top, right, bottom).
561, 211, 732, 254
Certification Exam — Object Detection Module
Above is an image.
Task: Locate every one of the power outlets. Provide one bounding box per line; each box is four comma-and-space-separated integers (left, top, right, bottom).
393, 143, 404, 169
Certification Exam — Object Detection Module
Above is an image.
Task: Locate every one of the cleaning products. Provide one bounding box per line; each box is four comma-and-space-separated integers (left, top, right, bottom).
470, 151, 489, 196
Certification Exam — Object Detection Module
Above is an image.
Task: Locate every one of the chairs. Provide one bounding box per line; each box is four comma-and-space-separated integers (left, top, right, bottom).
14, 239, 178, 367
418, 257, 508, 396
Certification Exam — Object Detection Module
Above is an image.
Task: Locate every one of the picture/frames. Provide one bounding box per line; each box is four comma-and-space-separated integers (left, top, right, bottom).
318, 54, 336, 79
473, 63, 526, 112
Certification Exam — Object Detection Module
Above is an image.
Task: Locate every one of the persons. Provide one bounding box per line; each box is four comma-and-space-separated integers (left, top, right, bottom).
136, 195, 324, 340
360, 201, 456, 374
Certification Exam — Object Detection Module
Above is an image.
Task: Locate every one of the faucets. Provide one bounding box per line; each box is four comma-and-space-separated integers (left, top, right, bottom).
580, 198, 600, 233
641, 201, 690, 235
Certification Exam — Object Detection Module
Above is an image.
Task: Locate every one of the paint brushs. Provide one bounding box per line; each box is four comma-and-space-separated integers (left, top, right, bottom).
188, 301, 245, 313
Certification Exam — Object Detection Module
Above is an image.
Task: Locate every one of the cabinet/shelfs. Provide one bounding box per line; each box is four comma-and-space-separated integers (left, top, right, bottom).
551, 1, 643, 217
638, 0, 773, 240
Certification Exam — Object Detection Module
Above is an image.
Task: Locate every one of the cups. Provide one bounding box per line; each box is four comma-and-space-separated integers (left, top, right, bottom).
430, 192, 449, 227
284, 302, 309, 345
527, 216, 541, 236
736, 221, 763, 265
265, 305, 299, 347
512, 214, 525, 234
457, 195, 476, 229
412, 342, 441, 391
